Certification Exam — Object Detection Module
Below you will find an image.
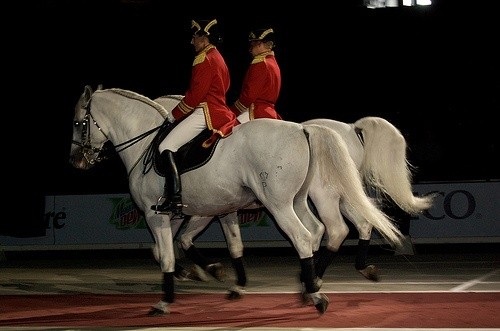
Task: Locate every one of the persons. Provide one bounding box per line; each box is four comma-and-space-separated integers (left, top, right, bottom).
151, 18, 236, 214
229, 26, 283, 123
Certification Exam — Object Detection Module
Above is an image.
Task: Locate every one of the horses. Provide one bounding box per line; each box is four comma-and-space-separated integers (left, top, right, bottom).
153, 94, 437, 292
69, 82, 407, 317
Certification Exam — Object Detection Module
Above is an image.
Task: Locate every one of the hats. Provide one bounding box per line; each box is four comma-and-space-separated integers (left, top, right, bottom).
192, 20, 223, 43
250, 28, 276, 41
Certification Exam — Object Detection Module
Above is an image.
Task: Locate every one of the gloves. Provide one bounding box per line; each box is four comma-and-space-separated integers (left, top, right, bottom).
167, 112, 176, 123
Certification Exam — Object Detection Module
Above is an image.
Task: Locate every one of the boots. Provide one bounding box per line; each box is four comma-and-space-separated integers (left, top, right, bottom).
150, 151, 184, 215
